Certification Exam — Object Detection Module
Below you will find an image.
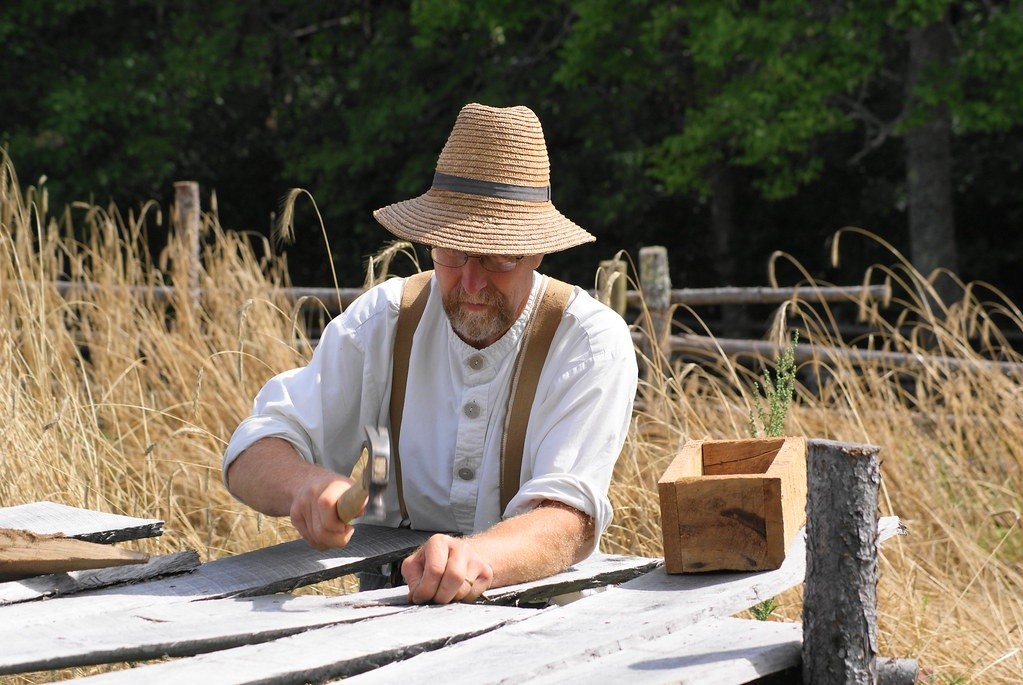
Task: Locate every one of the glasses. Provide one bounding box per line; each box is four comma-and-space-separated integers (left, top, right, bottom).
426, 245, 525, 273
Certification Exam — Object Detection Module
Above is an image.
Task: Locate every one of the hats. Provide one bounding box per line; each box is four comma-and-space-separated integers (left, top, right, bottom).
372, 103, 596, 257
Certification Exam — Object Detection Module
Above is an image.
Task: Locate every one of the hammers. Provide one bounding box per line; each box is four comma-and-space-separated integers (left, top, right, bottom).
336, 425, 391, 524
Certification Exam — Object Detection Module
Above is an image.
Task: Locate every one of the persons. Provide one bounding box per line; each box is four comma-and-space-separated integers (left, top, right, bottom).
220, 100, 639, 607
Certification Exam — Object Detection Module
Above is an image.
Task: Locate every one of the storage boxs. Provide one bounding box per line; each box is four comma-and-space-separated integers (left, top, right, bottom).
657, 435, 807, 574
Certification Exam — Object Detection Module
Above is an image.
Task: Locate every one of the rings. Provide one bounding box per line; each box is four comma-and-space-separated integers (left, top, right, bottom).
464, 576, 474, 586
